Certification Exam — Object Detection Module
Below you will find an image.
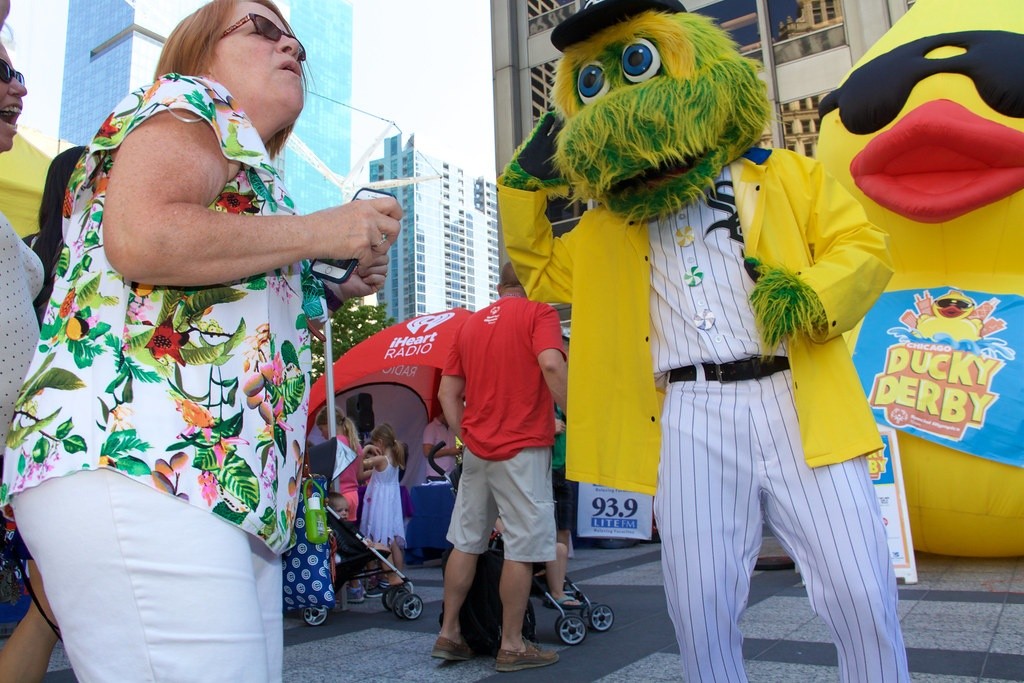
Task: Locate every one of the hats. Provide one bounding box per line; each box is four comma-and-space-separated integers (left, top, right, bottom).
550, 0, 688, 51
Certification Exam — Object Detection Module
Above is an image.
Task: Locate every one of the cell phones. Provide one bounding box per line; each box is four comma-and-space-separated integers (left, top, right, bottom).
310, 187, 395, 284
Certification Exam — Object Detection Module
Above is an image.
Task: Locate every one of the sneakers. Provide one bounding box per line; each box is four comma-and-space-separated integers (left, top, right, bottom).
494, 637, 559, 672
431, 635, 472, 662
346, 585, 365, 604
366, 583, 390, 598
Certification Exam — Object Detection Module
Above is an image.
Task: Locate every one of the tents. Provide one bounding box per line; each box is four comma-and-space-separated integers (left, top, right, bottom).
303, 308, 576, 560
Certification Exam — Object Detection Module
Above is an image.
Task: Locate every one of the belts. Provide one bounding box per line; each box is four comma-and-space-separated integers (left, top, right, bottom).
665, 354, 791, 384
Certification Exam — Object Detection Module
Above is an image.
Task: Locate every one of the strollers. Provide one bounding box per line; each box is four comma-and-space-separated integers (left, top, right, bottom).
282, 475, 423, 627
421, 440, 615, 647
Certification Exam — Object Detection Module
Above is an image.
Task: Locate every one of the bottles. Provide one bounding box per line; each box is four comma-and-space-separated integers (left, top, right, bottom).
305, 497, 327, 544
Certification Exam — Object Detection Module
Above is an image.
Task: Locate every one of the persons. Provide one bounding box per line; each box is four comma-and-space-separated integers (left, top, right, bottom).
536, 336, 584, 543
495, 516, 587, 610
431, 262, 568, 672
0, 41, 88, 683
422, 410, 462, 483
316, 406, 414, 604
0, 0, 404, 683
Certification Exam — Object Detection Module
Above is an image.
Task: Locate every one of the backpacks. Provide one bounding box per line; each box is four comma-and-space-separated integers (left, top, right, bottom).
438, 547, 536, 659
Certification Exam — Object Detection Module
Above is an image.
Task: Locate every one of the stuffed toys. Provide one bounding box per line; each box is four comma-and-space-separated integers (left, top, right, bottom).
496, 0, 909, 683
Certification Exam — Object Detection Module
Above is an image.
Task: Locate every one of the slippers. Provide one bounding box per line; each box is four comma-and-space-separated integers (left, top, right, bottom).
543, 596, 584, 609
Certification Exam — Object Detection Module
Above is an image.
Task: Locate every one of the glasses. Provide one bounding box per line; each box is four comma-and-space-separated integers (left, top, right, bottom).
0, 59, 25, 86
218, 13, 306, 61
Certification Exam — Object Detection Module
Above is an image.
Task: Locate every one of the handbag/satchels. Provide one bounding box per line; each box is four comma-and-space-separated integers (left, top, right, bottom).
282, 480, 336, 616
